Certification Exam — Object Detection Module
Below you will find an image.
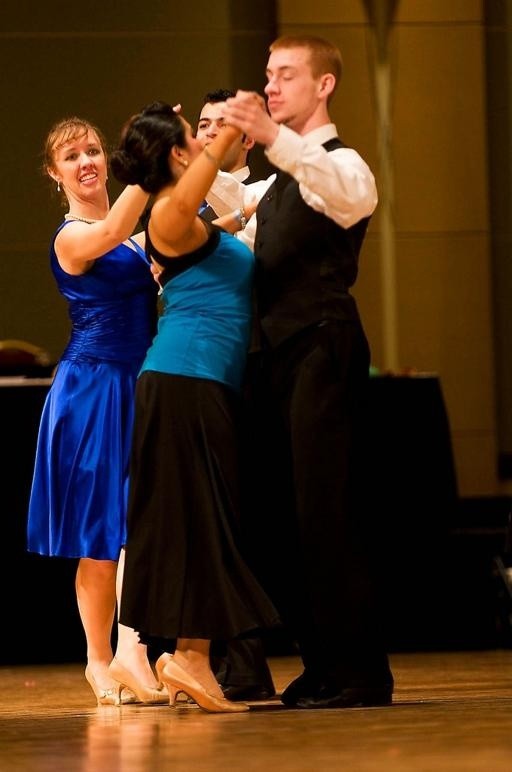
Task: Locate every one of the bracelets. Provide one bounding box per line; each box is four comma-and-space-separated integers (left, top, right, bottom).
234, 208, 247, 230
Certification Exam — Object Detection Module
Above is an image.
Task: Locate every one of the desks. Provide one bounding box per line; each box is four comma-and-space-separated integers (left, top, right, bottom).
0, 375, 468, 667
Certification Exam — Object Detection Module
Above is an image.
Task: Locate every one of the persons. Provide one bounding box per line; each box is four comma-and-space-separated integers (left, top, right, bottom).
151, 34, 392, 708
108, 100, 259, 713
150, 91, 275, 702
28, 102, 190, 708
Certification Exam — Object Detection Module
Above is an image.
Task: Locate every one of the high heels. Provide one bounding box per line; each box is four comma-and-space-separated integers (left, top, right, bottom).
85, 651, 250, 712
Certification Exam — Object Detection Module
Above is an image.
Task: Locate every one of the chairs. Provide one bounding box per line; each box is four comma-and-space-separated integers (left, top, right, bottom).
0, 338, 51, 376
